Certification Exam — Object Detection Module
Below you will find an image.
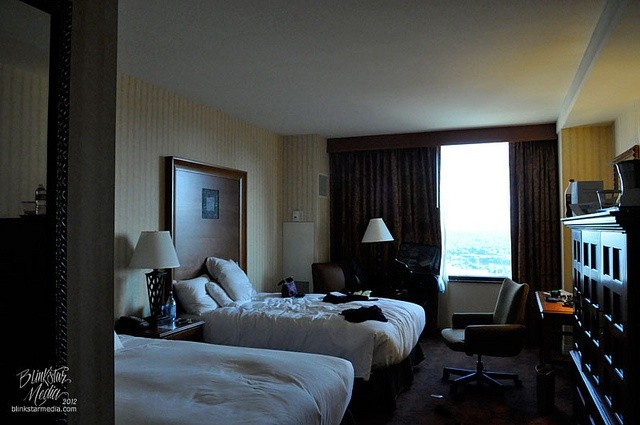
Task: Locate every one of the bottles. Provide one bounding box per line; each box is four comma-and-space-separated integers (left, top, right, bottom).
166, 291, 177, 330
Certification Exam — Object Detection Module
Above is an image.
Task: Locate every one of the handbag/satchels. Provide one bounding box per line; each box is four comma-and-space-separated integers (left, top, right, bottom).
278, 277, 298, 297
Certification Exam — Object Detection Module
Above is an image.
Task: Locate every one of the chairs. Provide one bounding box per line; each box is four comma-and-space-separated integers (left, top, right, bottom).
311, 262, 346, 295
440, 278, 534, 405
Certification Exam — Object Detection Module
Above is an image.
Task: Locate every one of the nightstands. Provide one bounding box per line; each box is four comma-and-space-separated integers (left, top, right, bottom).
144, 315, 207, 346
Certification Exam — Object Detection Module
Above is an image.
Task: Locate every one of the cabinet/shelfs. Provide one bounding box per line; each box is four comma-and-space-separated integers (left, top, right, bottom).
1, 218, 50, 425
560, 160, 639, 424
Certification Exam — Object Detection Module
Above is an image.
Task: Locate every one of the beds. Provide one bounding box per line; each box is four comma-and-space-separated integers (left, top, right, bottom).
166, 156, 428, 382
116, 337, 357, 425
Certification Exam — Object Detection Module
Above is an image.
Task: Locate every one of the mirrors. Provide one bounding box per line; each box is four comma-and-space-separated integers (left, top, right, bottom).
2, 0, 73, 423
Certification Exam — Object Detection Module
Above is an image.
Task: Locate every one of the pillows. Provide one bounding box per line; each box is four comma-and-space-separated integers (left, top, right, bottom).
207, 258, 255, 298
114, 332, 121, 352
204, 281, 233, 308
173, 274, 222, 319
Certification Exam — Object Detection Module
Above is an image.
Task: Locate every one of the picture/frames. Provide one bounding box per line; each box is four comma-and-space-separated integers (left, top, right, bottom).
317, 172, 329, 197
611, 145, 640, 209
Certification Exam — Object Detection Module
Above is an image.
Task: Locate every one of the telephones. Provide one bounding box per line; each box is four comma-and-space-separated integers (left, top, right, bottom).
119, 314, 149, 330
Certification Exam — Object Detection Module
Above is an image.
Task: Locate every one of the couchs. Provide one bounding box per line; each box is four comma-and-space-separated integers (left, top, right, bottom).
397, 242, 441, 334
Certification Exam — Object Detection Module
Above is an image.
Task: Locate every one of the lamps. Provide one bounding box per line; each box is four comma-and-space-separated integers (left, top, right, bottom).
129, 230, 183, 329
361, 218, 395, 293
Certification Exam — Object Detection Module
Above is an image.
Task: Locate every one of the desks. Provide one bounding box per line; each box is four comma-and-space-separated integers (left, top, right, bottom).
356, 268, 411, 297
534, 289, 579, 380
